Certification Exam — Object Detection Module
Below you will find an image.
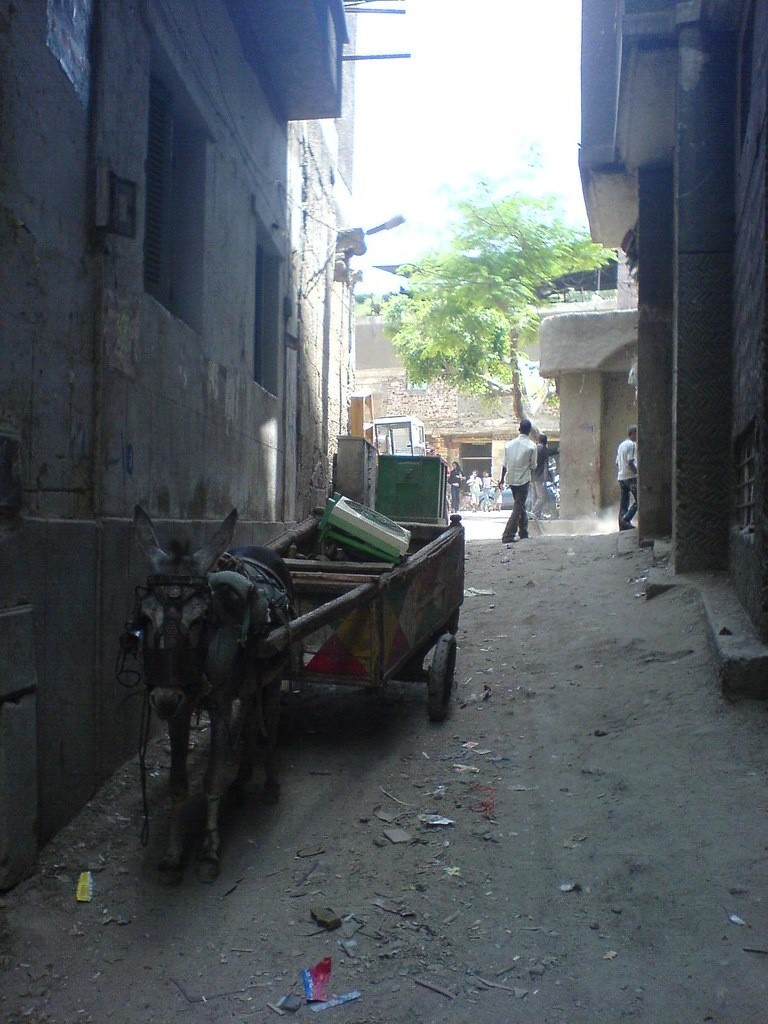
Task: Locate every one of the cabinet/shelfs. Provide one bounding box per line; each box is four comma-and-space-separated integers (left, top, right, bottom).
350, 393, 375, 446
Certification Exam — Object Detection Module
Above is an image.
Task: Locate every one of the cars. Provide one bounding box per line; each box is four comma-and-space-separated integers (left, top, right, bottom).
477, 477, 559, 512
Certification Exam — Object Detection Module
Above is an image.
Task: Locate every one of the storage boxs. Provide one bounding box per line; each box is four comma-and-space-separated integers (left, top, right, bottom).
374, 455, 448, 524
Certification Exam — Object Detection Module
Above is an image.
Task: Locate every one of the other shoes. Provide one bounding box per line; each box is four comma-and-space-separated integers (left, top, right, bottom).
519, 532, 528, 539
502, 536, 518, 543
620, 518, 635, 530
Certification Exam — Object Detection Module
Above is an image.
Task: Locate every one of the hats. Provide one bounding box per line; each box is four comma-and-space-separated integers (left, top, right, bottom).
628, 425, 637, 434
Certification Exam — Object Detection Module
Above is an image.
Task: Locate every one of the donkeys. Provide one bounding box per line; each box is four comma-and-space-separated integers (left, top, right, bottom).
132, 502, 298, 888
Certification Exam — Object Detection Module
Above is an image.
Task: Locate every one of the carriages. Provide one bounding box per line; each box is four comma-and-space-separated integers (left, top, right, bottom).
113, 503, 466, 888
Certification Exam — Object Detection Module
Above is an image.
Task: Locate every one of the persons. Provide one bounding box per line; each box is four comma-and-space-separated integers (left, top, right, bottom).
497, 420, 538, 543
447, 434, 560, 518
616, 423, 638, 532
